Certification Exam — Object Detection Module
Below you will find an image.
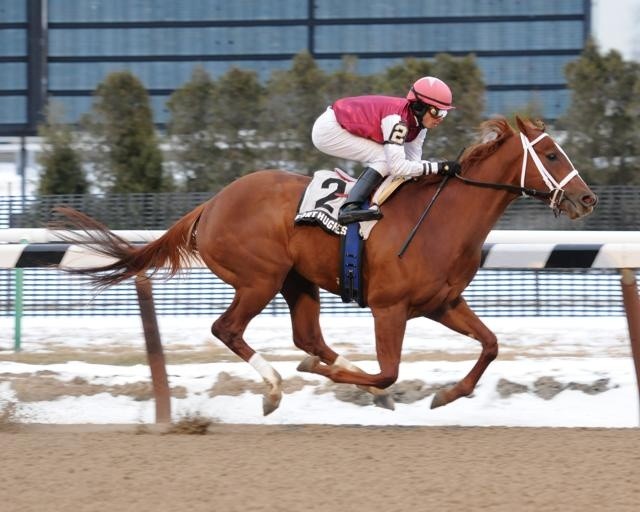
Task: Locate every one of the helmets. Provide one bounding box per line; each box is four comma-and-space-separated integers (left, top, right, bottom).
407, 76, 456, 111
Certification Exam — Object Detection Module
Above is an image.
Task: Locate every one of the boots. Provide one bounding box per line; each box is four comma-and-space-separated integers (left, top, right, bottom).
338, 166, 383, 224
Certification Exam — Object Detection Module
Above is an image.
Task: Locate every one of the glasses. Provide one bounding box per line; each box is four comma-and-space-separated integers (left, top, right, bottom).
410, 85, 448, 118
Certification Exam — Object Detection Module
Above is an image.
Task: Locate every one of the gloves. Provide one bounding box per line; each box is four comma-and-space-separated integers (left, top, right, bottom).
438, 161, 462, 177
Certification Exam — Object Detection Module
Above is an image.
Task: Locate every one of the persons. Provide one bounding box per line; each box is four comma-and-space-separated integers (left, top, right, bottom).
311, 76, 463, 228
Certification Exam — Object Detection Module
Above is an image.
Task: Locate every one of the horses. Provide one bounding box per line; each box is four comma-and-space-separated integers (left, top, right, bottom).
46, 113, 599, 417
